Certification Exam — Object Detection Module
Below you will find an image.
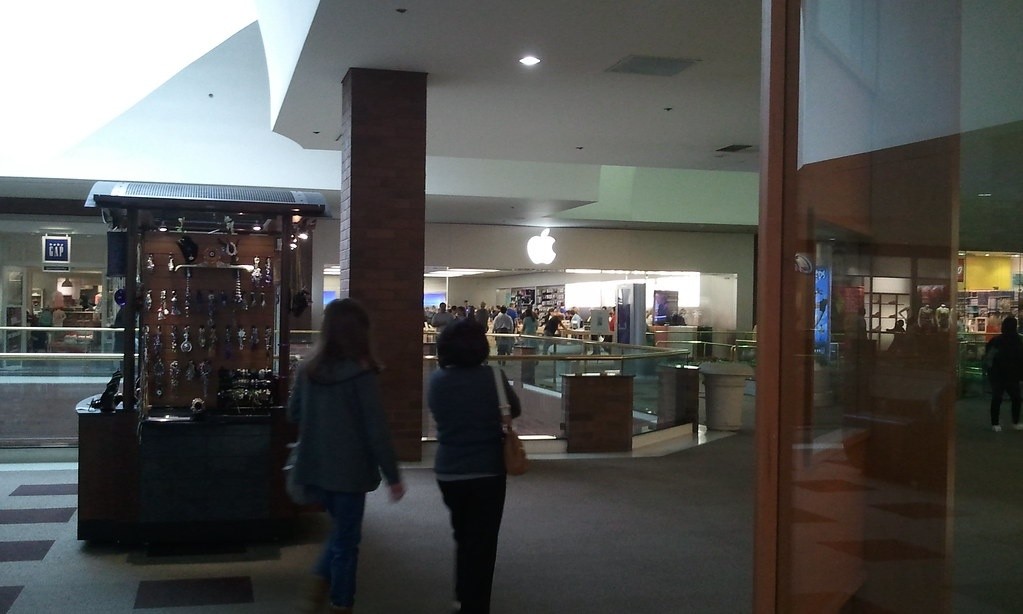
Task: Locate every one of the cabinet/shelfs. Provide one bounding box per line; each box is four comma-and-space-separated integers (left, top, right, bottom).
865, 292, 911, 333
0, 266, 101, 368
508, 281, 566, 313
959, 291, 1015, 338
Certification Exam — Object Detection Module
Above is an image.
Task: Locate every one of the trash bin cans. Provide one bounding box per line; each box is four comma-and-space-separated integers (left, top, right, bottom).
702, 370, 747, 432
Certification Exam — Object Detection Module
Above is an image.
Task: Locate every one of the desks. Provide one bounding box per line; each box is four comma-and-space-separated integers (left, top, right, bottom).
559, 372, 636, 453
659, 364, 701, 434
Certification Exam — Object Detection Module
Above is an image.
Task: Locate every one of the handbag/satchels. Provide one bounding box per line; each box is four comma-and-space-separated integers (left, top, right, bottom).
503, 431, 530, 476
982, 336, 1000, 376
284, 441, 321, 504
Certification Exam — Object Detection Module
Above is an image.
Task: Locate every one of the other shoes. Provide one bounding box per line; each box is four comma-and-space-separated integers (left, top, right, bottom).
992, 425, 1002, 432
329, 606, 353, 614
309, 575, 330, 602
1013, 423, 1023, 430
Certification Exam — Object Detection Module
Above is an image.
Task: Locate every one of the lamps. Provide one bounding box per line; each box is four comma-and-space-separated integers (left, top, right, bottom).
176, 235, 199, 263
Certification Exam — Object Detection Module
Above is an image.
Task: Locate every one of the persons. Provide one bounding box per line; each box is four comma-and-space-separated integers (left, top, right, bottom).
114, 289, 125, 353
285, 296, 406, 614
38, 309, 68, 327
427, 317, 523, 614
846, 308, 871, 367
985, 317, 1023, 432
917, 304, 951, 334
423, 290, 585, 366
895, 320, 906, 332
602, 306, 616, 331
